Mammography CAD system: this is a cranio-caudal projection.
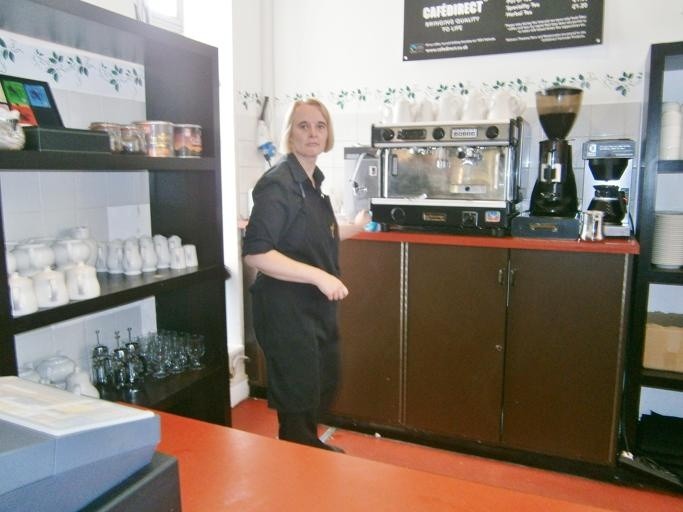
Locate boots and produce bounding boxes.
[276,409,346,455]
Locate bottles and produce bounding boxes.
[174,123,202,159]
[92,345,112,386]
[65,366,100,399]
[71,227,99,267]
[125,341,149,384]
[121,124,147,153]
[138,122,175,157]
[18,361,41,383]
[112,348,133,389]
[91,122,122,152]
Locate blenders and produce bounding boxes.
[530,87,583,217]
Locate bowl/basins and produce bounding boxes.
[660,101,683,161]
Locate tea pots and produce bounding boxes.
[168,235,186,268]
[106,239,122,274]
[122,239,142,277]
[52,236,89,267]
[8,272,38,317]
[96,241,107,273]
[33,265,69,308]
[184,243,199,266]
[12,240,52,272]
[153,234,169,269]
[139,234,157,273]
[66,259,101,300]
[6,249,16,274]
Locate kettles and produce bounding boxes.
[36,351,76,384]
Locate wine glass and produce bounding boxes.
[139,331,206,380]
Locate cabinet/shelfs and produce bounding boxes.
[619,39,683,489]
[239,227,401,440]
[401,244,633,481]
[0,0,232,430]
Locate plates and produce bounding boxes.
[652,210,683,270]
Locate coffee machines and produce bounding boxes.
[581,139,633,237]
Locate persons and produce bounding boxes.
[242,97,372,454]
